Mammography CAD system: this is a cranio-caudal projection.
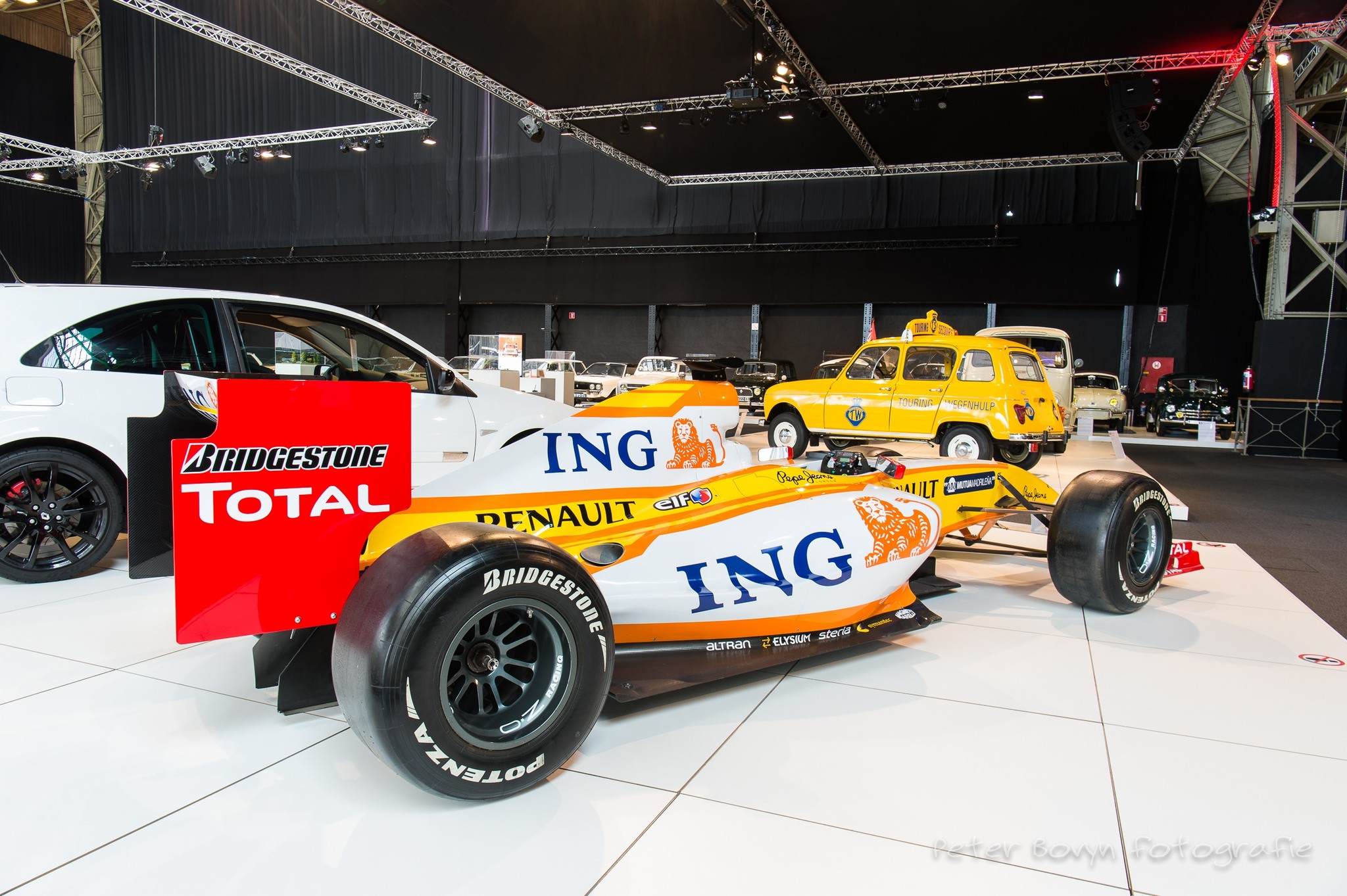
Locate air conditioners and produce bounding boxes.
[907,91,924,111]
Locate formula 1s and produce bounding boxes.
[124,379,1206,802]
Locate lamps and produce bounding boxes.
[863,94,888,115]
[1244,41,1267,77]
[238,148,249,163]
[226,150,237,164]
[778,102,794,119]
[339,134,384,153]
[754,32,809,95]
[805,99,825,120]
[642,115,657,130]
[1251,206,1278,221]
[517,114,545,143]
[700,109,714,128]
[193,151,217,179]
[937,89,949,109]
[1275,42,1293,67]
[254,144,291,158]
[727,107,751,127]
[1028,81,1044,99]
[619,115,630,134]
[679,109,694,126]
[561,120,575,136]
[0,125,175,192]
[418,129,437,145]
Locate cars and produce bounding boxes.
[1146,372,1236,440]
[762,309,1127,472]
[0,279,595,588]
[357,347,798,411]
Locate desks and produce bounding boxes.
[1232,397,1342,460]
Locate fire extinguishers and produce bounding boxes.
[1139,401,1146,417]
[1243,366,1254,390]
